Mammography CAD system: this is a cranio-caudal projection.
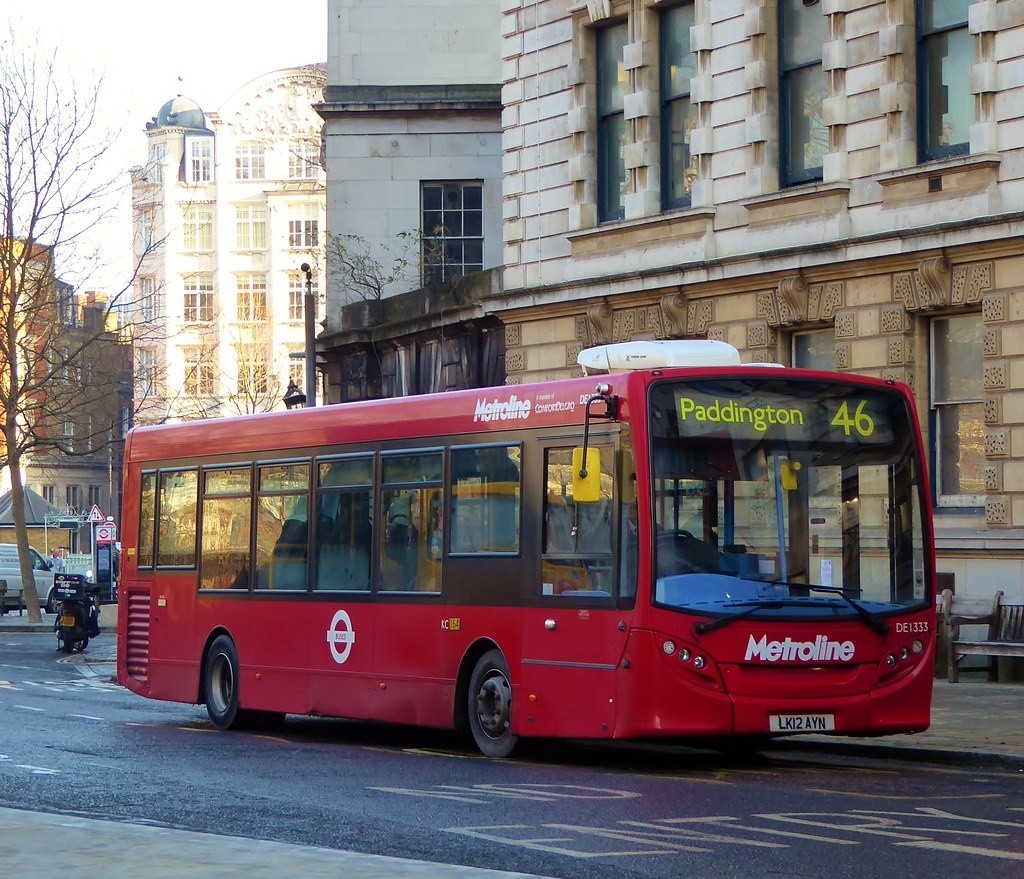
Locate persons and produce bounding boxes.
[44,547,63,574]
[609,498,664,550]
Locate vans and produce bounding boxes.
[0,542,65,614]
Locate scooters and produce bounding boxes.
[53,579,100,652]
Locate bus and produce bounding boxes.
[114,339,939,764]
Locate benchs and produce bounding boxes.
[945,590,1024,684]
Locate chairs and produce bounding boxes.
[248,511,423,592]
